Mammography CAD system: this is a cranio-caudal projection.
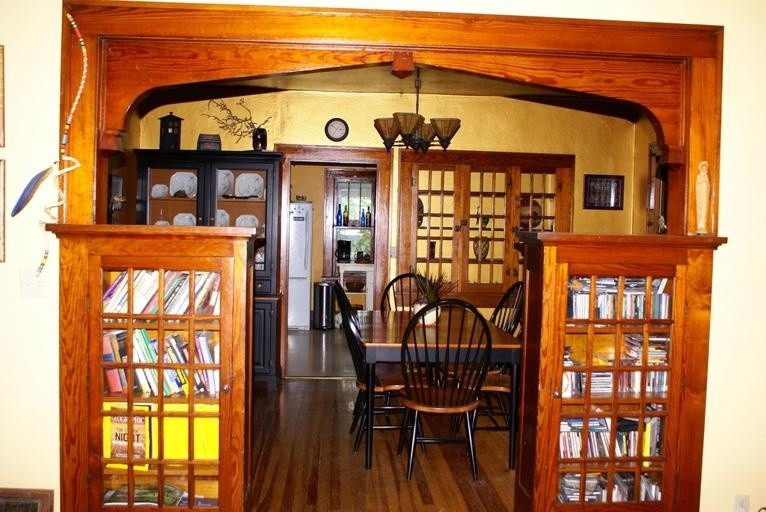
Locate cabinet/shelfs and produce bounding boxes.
[514,229,728,511]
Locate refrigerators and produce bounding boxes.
[288,201,313,331]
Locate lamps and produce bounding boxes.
[373,68,461,153]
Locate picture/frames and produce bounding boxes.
[583,174,624,211]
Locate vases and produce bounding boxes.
[252,128,267,150]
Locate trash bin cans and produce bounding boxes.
[313,282,336,331]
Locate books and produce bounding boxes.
[95,262,219,507]
[556,270,668,504]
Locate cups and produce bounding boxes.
[354,251,363,261]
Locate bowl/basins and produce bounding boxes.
[296,195,307,201]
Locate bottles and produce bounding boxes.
[366,206,371,227]
[343,205,349,226]
[364,255,370,261]
[337,204,342,225]
[361,208,365,227]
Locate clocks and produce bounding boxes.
[324,118,349,142]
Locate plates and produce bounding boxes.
[150,183,168,199]
[169,172,197,198]
[235,214,259,227]
[235,173,264,200]
[216,169,234,197]
[216,209,229,227]
[155,220,170,225]
[173,213,196,226]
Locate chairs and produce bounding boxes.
[332,273,524,482]
